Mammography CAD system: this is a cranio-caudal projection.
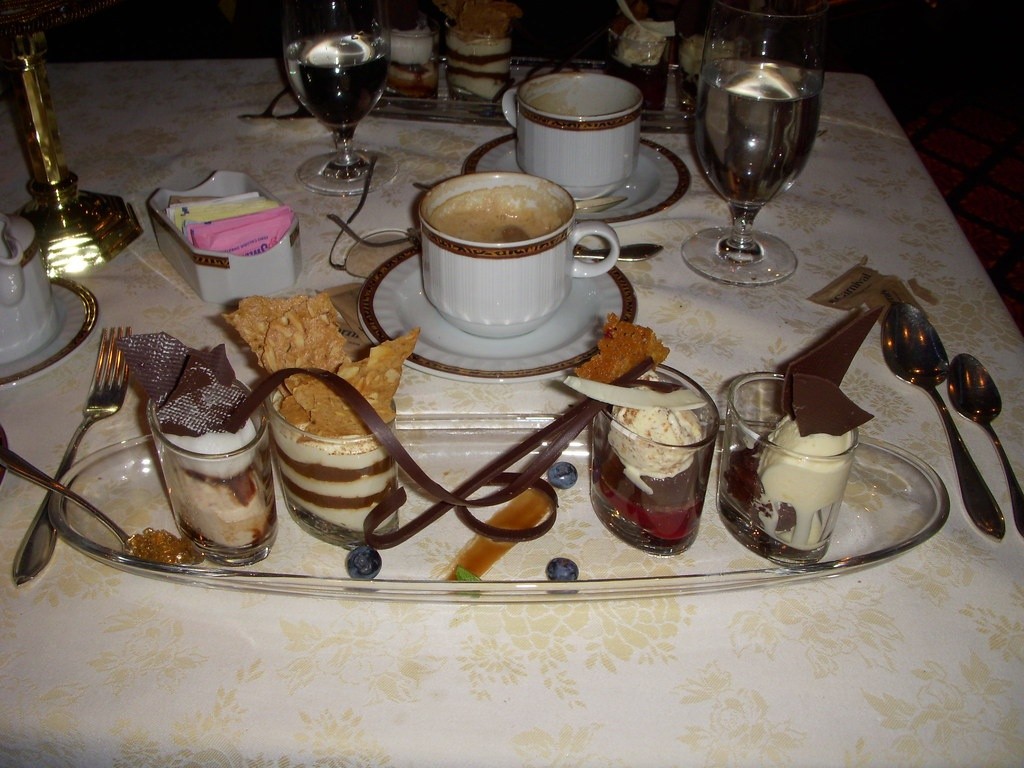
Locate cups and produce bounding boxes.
[714,372,859,567]
[606,26,673,110]
[265,393,399,548]
[589,362,719,556]
[385,21,440,97]
[0,210,62,366]
[501,70,641,202]
[417,174,622,338]
[444,16,512,103]
[146,378,277,558]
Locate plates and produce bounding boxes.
[358,246,638,384]
[0,278,99,392]
[461,132,691,225]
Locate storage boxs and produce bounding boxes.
[147,170,300,304]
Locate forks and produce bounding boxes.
[12,326,134,587]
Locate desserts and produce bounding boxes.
[376,0,743,113]
[116,300,887,567]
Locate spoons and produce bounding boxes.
[0,446,204,565]
[880,301,1004,541]
[238,86,316,122]
[947,353,1024,538]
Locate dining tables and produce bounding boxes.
[0,59,1024,768]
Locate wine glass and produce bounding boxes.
[682,0,834,287]
[281,0,400,196]
